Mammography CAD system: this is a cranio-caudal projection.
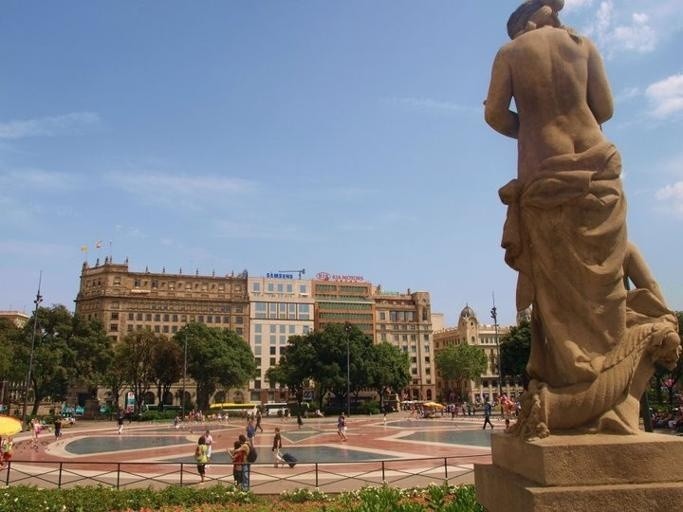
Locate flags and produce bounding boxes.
[80,245,87,253]
[95,241,101,248]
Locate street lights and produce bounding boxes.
[182,333,194,418]
[21,270,45,434]
[489,288,504,417]
[344,322,352,418]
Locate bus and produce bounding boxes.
[264,402,290,417]
[144,404,183,417]
[209,403,257,416]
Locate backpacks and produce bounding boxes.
[242,443,257,462]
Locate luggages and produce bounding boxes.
[279,451,297,468]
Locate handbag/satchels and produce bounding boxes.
[195,454,203,461]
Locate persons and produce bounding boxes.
[69,412,75,425]
[483,399,494,429]
[648,404,683,432]
[483,1,664,383]
[135,400,149,412]
[338,411,348,441]
[227,434,251,487]
[31,418,62,440]
[196,431,212,482]
[495,394,516,427]
[383,404,477,418]
[272,427,286,467]
[1,435,12,469]
[118,408,132,433]
[239,409,264,444]
[281,408,321,427]
[175,408,229,424]
